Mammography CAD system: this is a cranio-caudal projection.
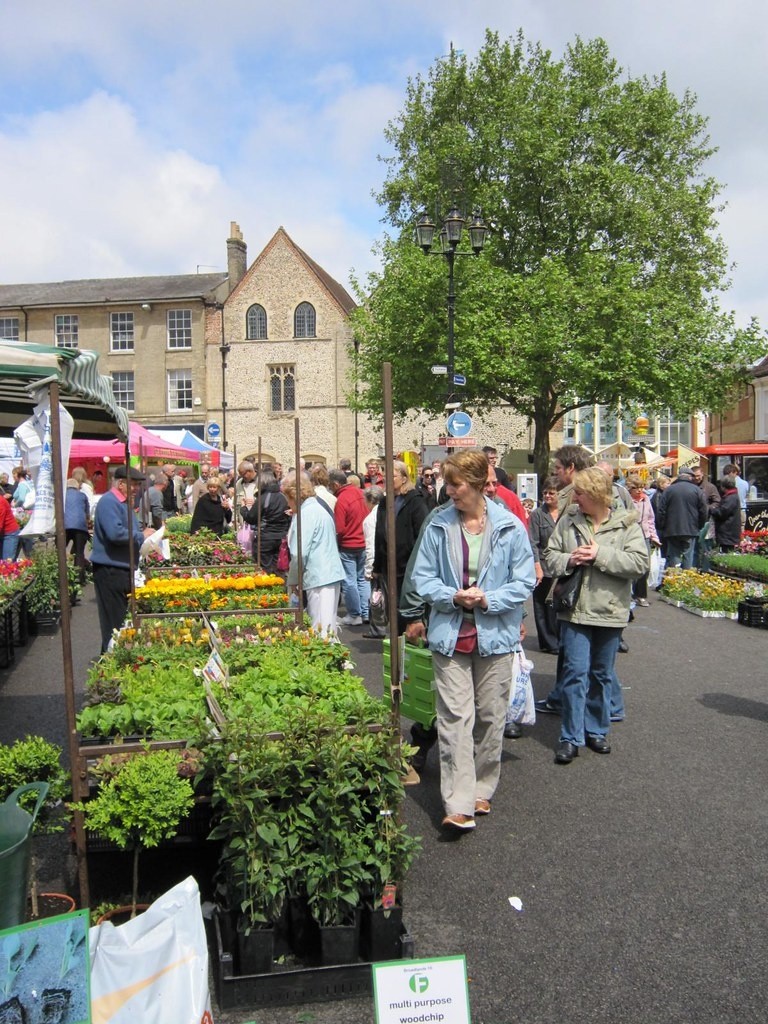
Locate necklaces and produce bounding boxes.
[461,499,486,535]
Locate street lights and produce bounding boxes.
[414,187,490,451]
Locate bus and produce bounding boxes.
[668,444,768,536]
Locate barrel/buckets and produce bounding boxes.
[0,781,50,930]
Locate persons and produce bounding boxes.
[0,445,750,777]
[410,449,537,827]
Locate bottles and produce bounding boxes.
[750,485,757,499]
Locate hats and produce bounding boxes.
[679,467,695,476]
[114,466,147,481]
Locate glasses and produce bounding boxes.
[542,491,557,495]
[633,486,644,490]
[423,474,432,478]
[488,456,498,459]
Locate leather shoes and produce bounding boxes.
[584,735,611,753]
[555,742,578,762]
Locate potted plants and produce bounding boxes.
[31,546,75,634]
[0,737,193,928]
[220,723,409,975]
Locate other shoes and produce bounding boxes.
[504,722,522,738]
[547,648,559,654]
[635,598,649,606]
[618,641,629,652]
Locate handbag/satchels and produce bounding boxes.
[646,545,666,588]
[505,642,536,726]
[705,515,716,539]
[367,575,389,637]
[552,526,584,611]
[277,538,291,571]
[237,520,254,555]
[22,482,35,508]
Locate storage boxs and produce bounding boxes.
[738,601,764,626]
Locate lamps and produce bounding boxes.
[142,303,151,313]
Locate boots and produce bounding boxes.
[407,722,438,769]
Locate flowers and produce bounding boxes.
[660,566,767,619]
[734,530,768,552]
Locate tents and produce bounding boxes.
[663,444,768,482]
[0,338,236,911]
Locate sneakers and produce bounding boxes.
[341,614,363,625]
[610,705,624,721]
[534,700,562,714]
[441,812,476,828]
[474,798,490,813]
[363,617,369,623]
